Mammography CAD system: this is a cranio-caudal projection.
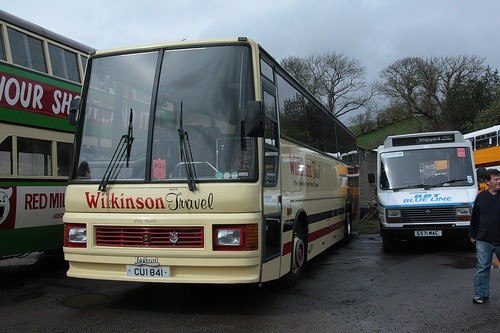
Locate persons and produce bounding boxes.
[468,169,500,303]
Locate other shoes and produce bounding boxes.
[492,259,499,268]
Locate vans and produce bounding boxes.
[368,131,478,249]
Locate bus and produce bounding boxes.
[63,36,358,290]
[0,12,95,260]
[462,124,500,194]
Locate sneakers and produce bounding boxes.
[472,295,489,304]
